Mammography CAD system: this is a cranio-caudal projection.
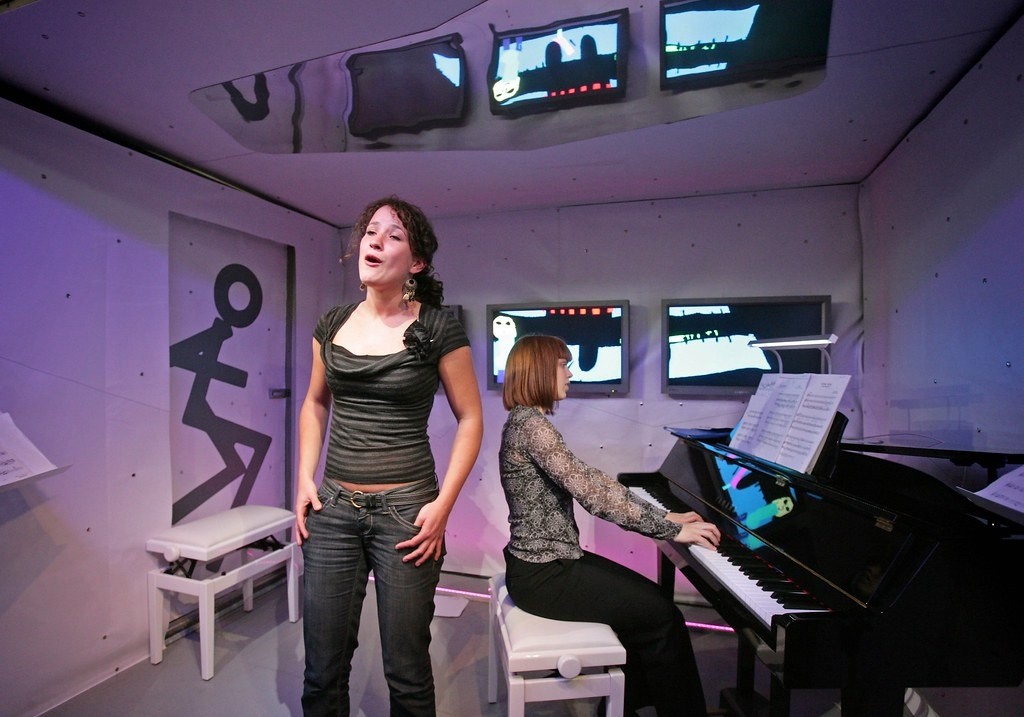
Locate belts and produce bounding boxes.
[322,478,440,508]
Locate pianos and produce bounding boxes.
[618,413,1024,717]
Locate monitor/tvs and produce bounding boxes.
[659,0,834,91]
[488,8,629,117]
[662,295,831,396]
[487,299,630,393]
[346,32,467,135]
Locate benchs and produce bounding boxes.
[145,505,300,680]
[487,571,627,717]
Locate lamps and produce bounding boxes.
[748,334,838,374]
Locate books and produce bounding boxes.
[728,373,853,474]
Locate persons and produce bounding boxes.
[291,198,485,717]
[498,335,722,717]
